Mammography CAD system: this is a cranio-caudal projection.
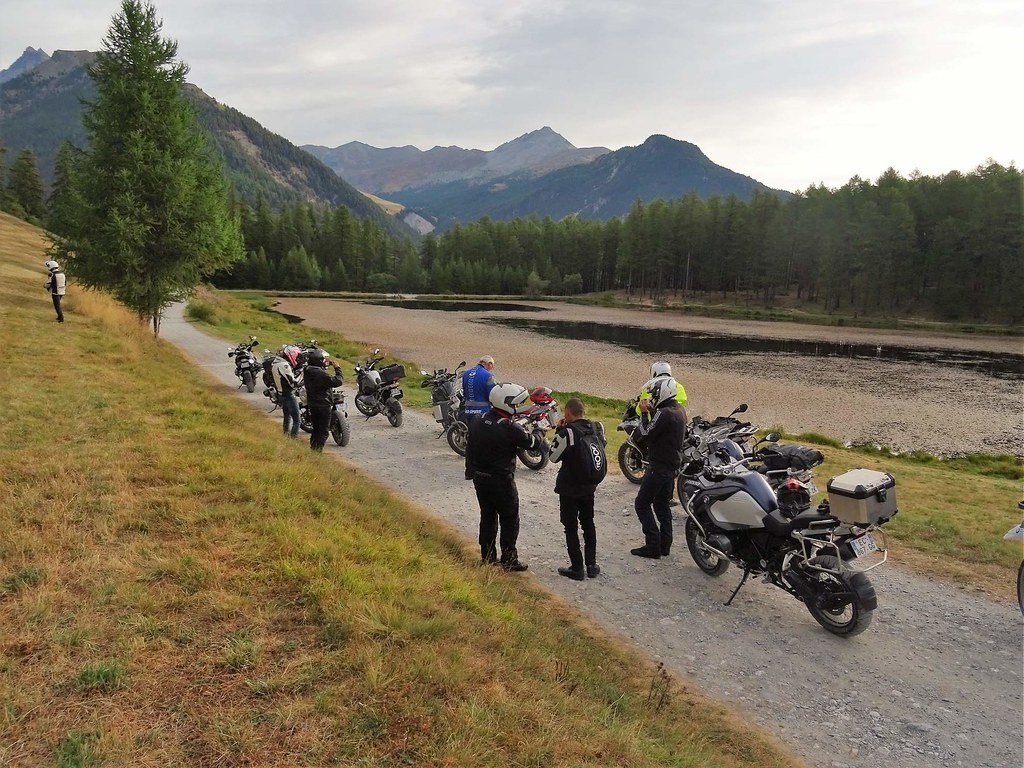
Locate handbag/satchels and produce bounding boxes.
[756,443,824,471]
[431,381,453,401]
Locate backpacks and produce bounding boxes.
[569,421,607,486]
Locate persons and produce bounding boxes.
[304,349,342,451]
[42,262,67,323]
[461,355,495,479]
[465,382,552,571]
[549,398,607,581]
[635,361,687,507]
[271,345,302,439]
[631,378,688,558]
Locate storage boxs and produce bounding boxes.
[434,401,449,423]
[827,468,898,528]
[379,364,405,381]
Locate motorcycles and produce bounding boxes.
[616,393,762,485]
[352,348,406,429]
[420,361,561,472]
[673,430,900,638]
[259,339,351,447]
[226,335,261,394]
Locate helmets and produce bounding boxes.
[647,377,677,409]
[46,261,59,271]
[530,386,555,405]
[489,382,529,414]
[307,349,329,367]
[777,479,811,517]
[650,361,672,378]
[285,346,302,367]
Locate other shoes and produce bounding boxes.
[661,544,670,555]
[558,566,585,580]
[587,564,600,577]
[668,499,678,506]
[482,559,501,564]
[503,561,528,571]
[631,545,661,558]
